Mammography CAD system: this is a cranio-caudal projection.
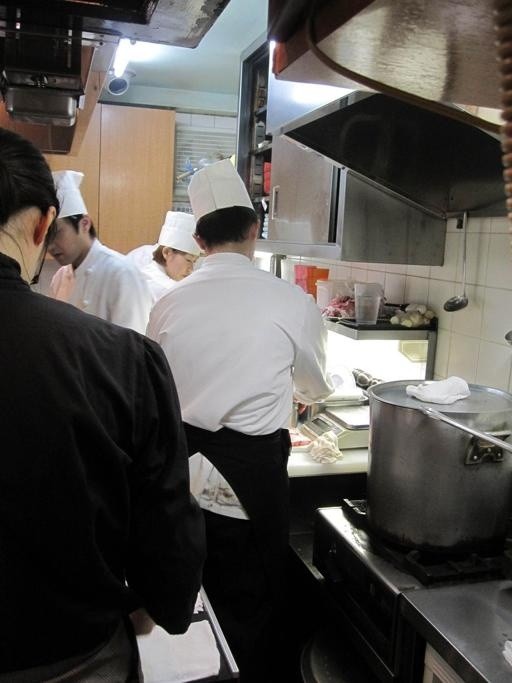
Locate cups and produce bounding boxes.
[353,282,381,326]
[305,266,330,301]
[293,264,317,293]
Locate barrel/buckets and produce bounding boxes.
[317,277,353,313]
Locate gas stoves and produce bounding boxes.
[342,498,512,589]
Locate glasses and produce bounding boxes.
[28,226,50,284]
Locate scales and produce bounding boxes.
[300,404,369,448]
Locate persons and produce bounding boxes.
[145,240,200,322]
[148,206,335,682]
[0,123,208,682]
[44,209,147,342]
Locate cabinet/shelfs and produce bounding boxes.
[44,101,177,260]
[233,29,447,266]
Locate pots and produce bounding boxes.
[362,378,511,557]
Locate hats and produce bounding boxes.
[49,167,89,218]
[187,156,256,222]
[159,208,204,259]
